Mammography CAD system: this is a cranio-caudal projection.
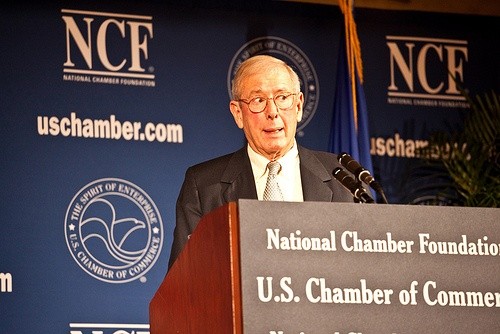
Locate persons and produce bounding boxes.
[167,56,373,272]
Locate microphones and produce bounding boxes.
[337,152,389,204]
[332,167,376,204]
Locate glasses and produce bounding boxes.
[234,93,299,114]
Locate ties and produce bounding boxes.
[263,162,284,201]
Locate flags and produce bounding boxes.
[325,0,376,200]
